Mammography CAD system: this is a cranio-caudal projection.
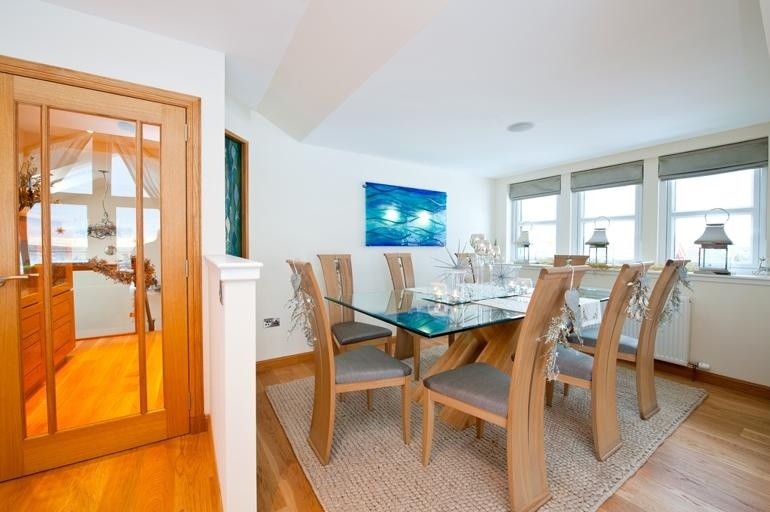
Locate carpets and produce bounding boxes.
[266,342,709,512]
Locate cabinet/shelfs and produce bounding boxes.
[21,264,76,402]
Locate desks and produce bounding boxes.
[321,277,614,442]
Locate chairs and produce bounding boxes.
[419,265,593,512]
[561,257,690,419]
[285,257,415,467]
[316,254,395,410]
[563,259,655,458]
[383,253,419,289]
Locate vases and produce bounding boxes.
[18,205,31,217]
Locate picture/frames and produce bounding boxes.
[225,130,249,260]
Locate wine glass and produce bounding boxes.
[469,233,500,266]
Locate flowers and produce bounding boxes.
[16,156,64,212]
[87,254,158,291]
